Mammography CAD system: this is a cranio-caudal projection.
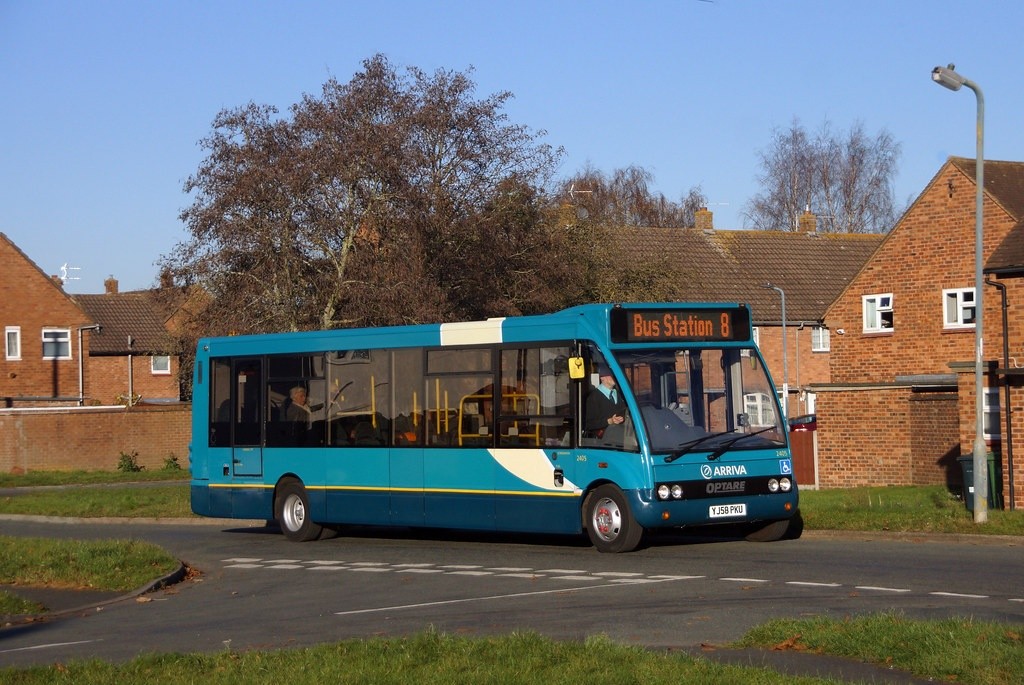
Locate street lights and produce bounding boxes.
[931,63,988,524]
[760,283,789,446]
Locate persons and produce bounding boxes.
[586,369,626,439]
[287,387,312,431]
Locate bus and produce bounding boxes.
[189,301,803,554]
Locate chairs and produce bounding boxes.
[217,398,479,448]
